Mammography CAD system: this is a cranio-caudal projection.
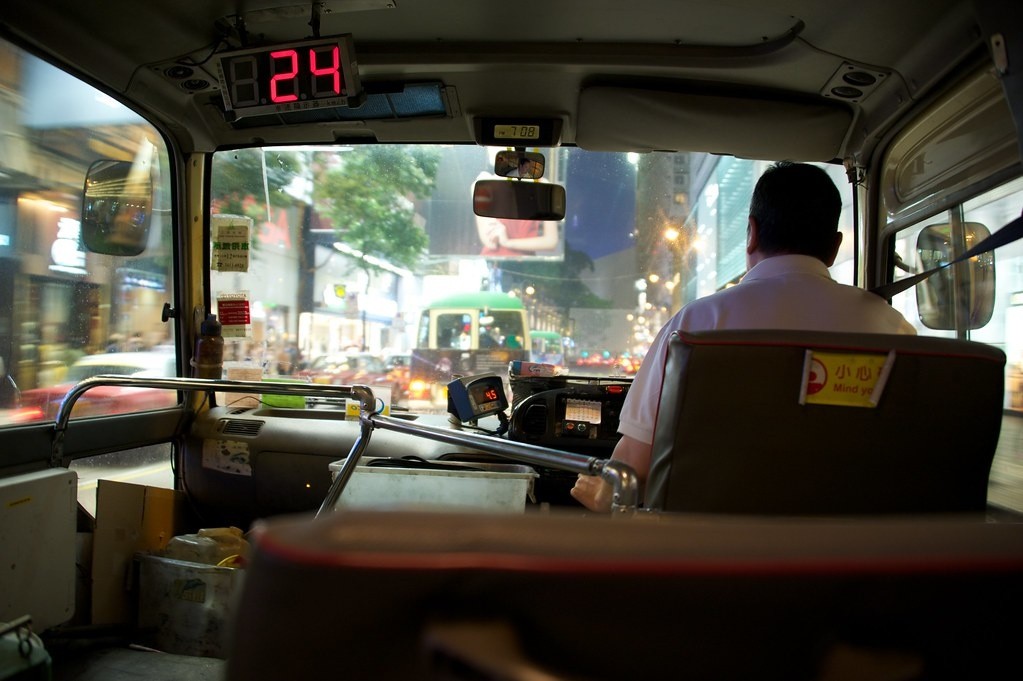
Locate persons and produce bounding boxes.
[472,147,559,256]
[568,161,916,514]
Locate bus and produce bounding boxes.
[415,297,530,384]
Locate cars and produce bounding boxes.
[385,354,437,400]
[296,352,399,405]
[18,352,179,423]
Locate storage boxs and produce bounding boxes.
[130,553,240,655]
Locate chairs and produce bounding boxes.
[219,508,1023,681]
[641,329,1007,519]
